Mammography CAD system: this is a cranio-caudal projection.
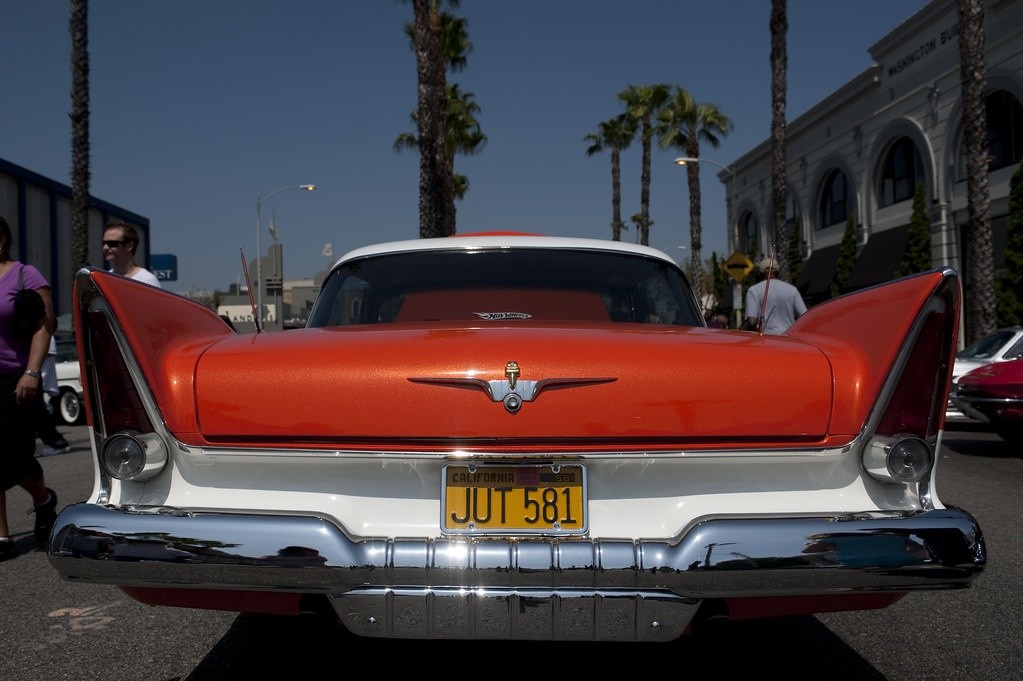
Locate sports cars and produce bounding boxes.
[48,233,988,643]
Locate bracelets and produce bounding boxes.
[25,370,40,378]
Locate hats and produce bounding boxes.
[758,258,780,275]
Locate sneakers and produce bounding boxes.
[0,536,18,562]
[26,487,58,542]
[41,438,71,456]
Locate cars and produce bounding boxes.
[952,325,1023,446]
[42,338,88,425]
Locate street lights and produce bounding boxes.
[256,184,319,333]
[673,156,742,332]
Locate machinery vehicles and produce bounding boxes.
[239,246,260,333]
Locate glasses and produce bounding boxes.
[102,240,128,248]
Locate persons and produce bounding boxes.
[0,216,71,561]
[705,309,729,329]
[102,222,161,288]
[743,258,807,336]
